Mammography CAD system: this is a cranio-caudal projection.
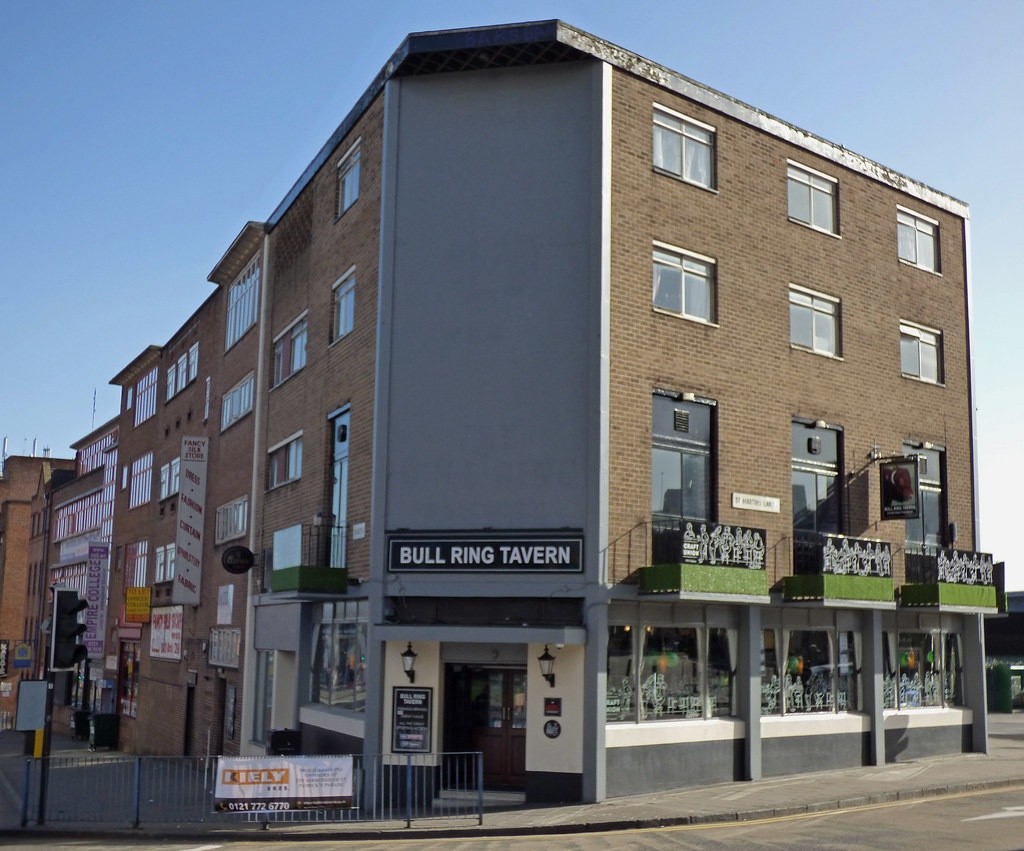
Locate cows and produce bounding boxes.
[882,465,913,506]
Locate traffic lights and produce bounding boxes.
[49,585,89,672]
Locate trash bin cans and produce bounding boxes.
[87,713,120,752]
[264,728,301,755]
[70,710,91,743]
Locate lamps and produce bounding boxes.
[538,644,555,687]
[401,641,417,683]
